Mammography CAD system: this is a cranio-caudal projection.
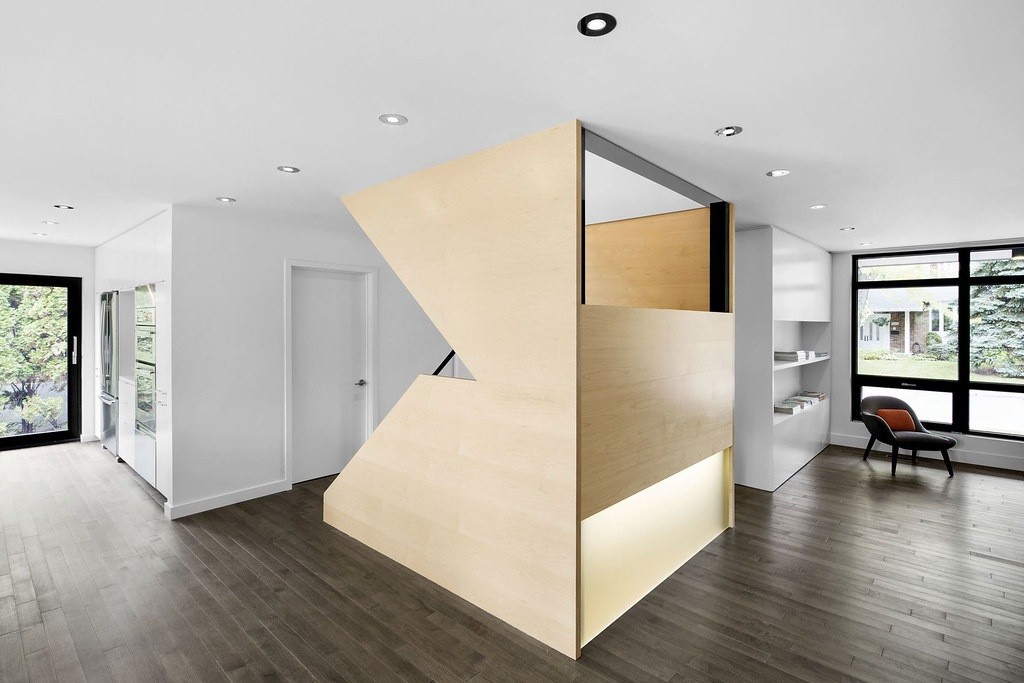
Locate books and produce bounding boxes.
[774,349,828,362]
[774,390,826,415]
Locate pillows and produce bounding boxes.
[878,409,916,431]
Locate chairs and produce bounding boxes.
[859,395,958,477]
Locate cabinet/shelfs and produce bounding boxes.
[734,226,833,493]
